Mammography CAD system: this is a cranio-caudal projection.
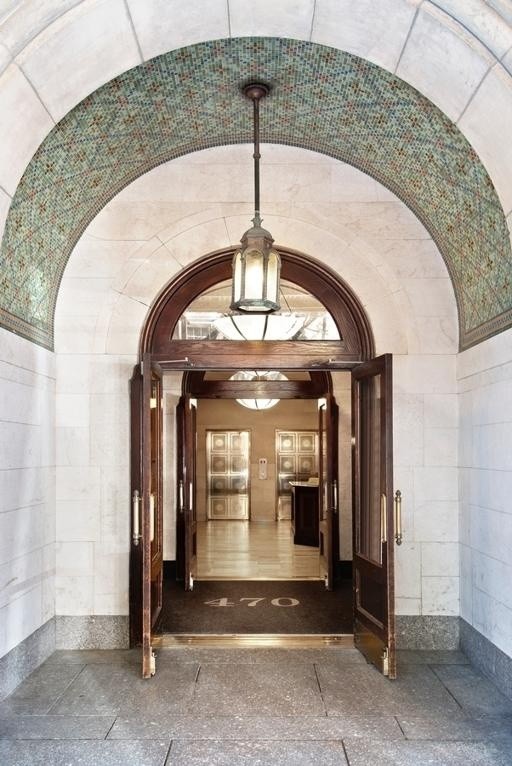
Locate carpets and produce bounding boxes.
[162,580,354,634]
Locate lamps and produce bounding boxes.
[230,82,282,315]
[211,280,307,412]
